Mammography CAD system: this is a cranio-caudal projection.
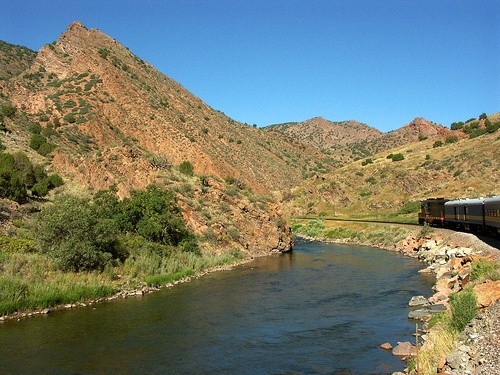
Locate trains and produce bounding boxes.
[416,196,500,239]
